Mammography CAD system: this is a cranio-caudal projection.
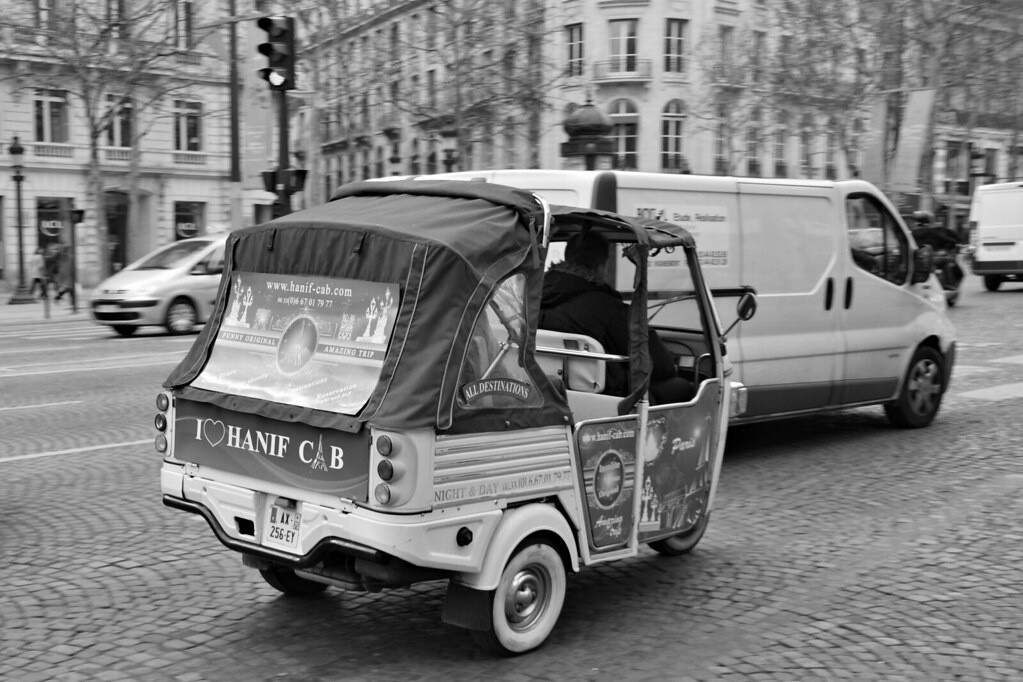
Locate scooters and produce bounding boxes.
[895,224,963,307]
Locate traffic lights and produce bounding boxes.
[257,18,295,89]
[283,169,303,188]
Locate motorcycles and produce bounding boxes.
[155,178,759,657]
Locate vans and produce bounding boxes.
[367,171,964,434]
[963,182,1023,290]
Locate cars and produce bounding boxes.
[847,227,907,273]
[92,234,228,336]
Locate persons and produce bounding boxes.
[910,210,954,290]
[28,248,50,299]
[526,227,685,413]
[932,213,966,288]
[48,246,78,311]
